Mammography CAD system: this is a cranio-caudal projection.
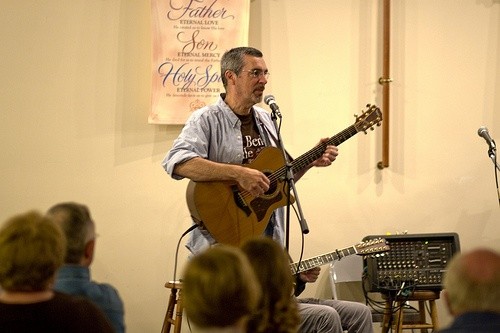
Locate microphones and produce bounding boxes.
[477,127,496,150]
[264,95,282,119]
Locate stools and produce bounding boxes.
[160,281,187,333]
[383,292,440,333]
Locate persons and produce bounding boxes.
[0,209,117,333]
[181,243,262,333]
[429,248,500,332]
[162,47,340,255]
[294,265,374,333]
[236,235,304,332]
[44,202,125,333]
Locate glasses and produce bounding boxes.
[233,69,269,77]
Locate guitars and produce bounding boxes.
[289,239,391,277]
[187,103,382,248]
